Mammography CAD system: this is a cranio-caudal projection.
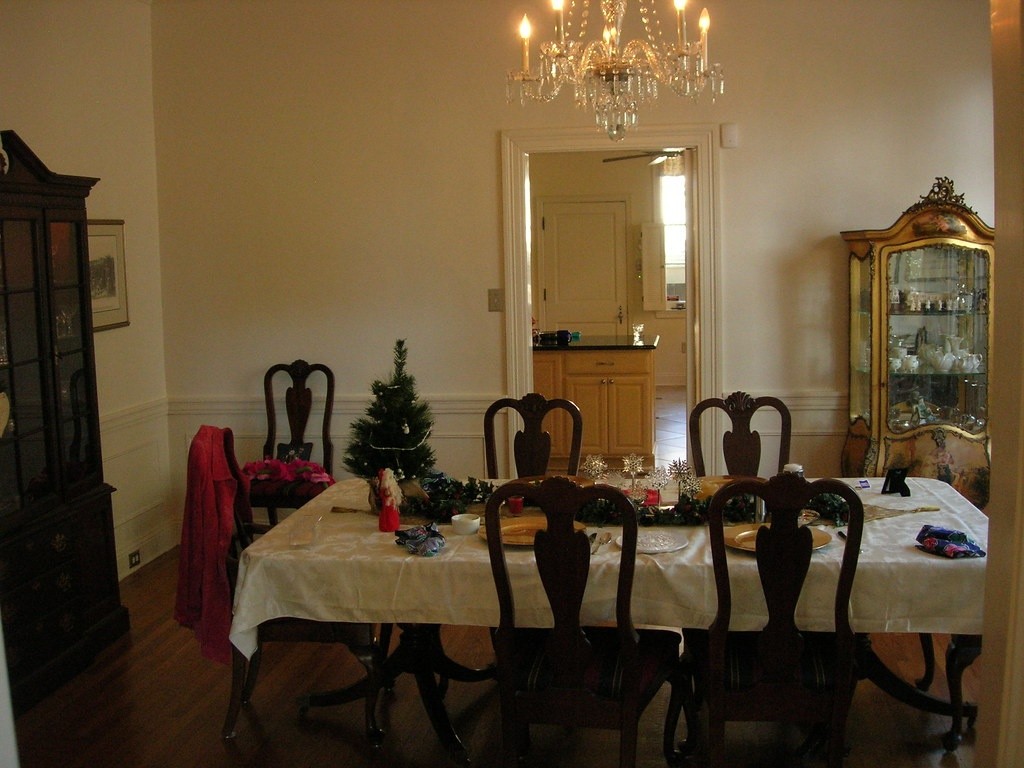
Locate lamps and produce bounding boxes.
[505,0,724,142]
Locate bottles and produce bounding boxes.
[783,463,804,479]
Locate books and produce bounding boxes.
[667,295,685,309]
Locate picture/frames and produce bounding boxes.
[87,219,130,332]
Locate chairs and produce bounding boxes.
[482,388,868,768]
[246,360,334,536]
[172,424,387,751]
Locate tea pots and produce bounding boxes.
[862,334,983,373]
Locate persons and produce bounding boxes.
[379,468,402,532]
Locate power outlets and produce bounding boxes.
[128,549,141,568]
[487,288,506,311]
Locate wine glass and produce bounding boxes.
[50,299,82,336]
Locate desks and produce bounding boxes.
[224,476,995,768]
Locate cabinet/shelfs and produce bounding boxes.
[838,174,996,511]
[0,128,134,723]
[533,349,656,483]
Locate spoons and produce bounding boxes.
[592,532,612,555]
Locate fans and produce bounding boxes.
[602,147,685,166]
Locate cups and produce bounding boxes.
[451,513,480,535]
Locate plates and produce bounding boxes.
[479,516,586,546]
[720,522,832,554]
[616,529,689,553]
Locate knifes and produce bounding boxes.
[586,533,597,546]
[838,529,848,541]
